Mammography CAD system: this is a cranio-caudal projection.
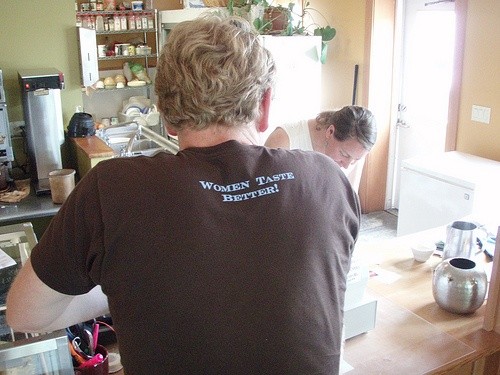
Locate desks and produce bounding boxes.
[0,179,63,223]
[344,219,500,375]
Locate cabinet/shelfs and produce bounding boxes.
[395,150,500,237]
[75,9,163,93]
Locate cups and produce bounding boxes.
[48,169,78,205]
[74,345,109,375]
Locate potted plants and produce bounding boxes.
[225,0,337,65]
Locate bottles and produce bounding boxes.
[82,12,154,31]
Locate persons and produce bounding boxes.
[263,106,378,194]
[6,10,360,375]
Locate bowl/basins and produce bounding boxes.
[412,244,434,264]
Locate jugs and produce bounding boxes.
[442,221,486,261]
